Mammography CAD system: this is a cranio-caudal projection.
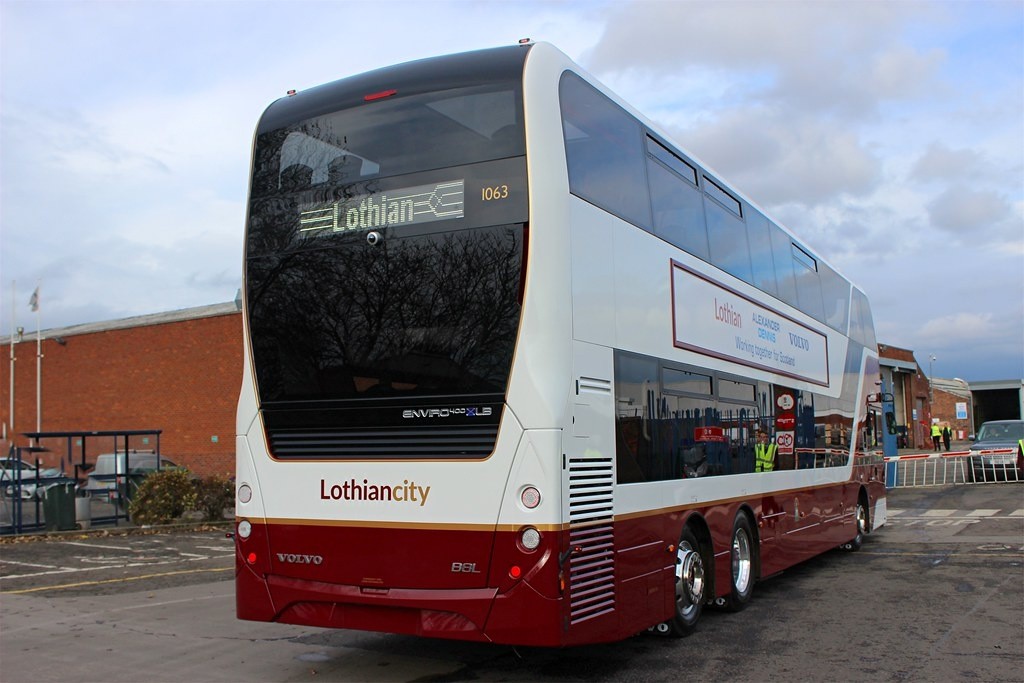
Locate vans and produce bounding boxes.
[94,448,179,502]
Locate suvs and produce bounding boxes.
[966,420,1024,482]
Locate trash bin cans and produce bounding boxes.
[40,477,82,534]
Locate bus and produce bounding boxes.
[235,39,897,648]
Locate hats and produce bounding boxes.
[944,422,947,424]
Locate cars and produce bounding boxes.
[0,457,43,490]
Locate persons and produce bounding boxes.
[985,427,1002,438]
[928,422,942,452]
[940,422,953,451]
[751,431,780,525]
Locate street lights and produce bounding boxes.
[929,354,936,421]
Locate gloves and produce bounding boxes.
[931,436,932,439]
